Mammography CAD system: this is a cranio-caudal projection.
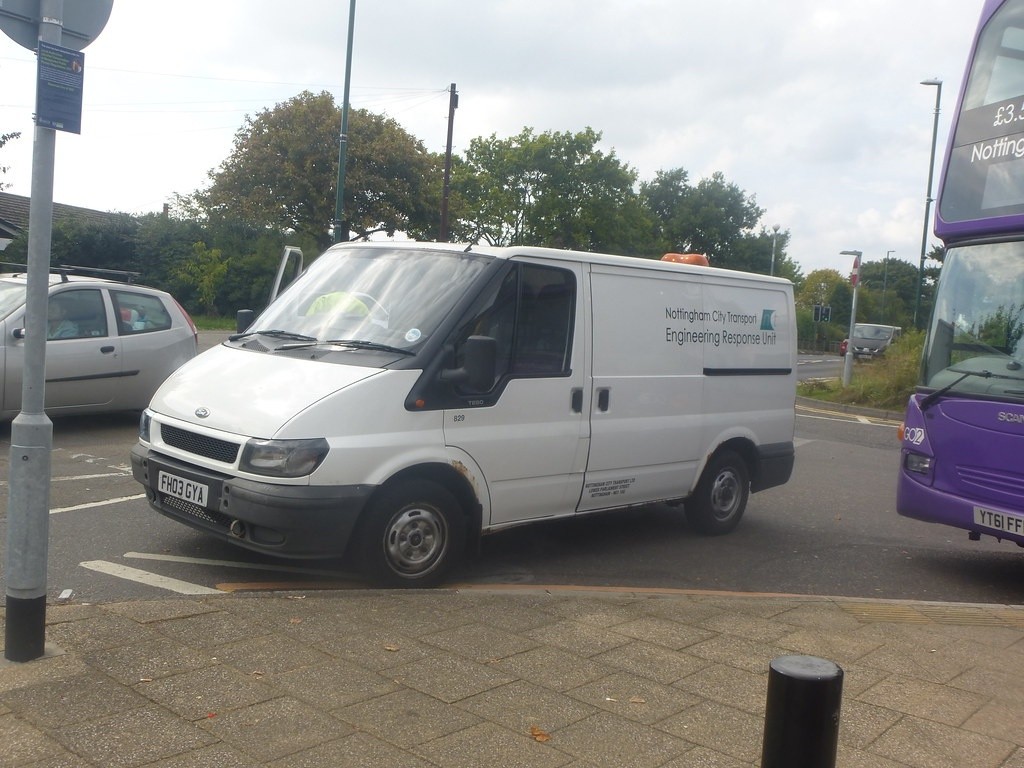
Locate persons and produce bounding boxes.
[46,297,81,339]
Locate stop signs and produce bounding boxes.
[849,257,860,287]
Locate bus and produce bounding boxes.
[897,0,1024,546]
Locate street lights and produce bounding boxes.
[839,249,863,391]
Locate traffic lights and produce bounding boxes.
[813,305,820,322]
[823,307,831,323]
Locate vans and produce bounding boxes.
[131,239,797,583]
[0,265,200,425]
[848,324,902,366]
